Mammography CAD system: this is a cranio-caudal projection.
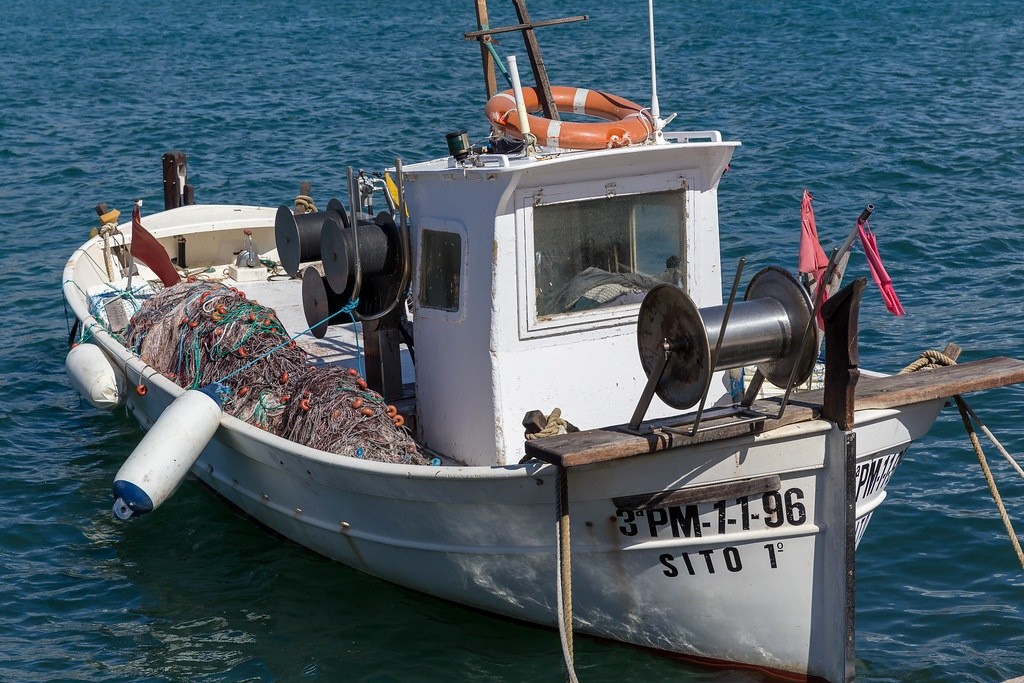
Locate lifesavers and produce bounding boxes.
[484,85,654,148]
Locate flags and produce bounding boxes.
[128,200,182,288]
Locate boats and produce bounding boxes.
[61,0,957,683]
